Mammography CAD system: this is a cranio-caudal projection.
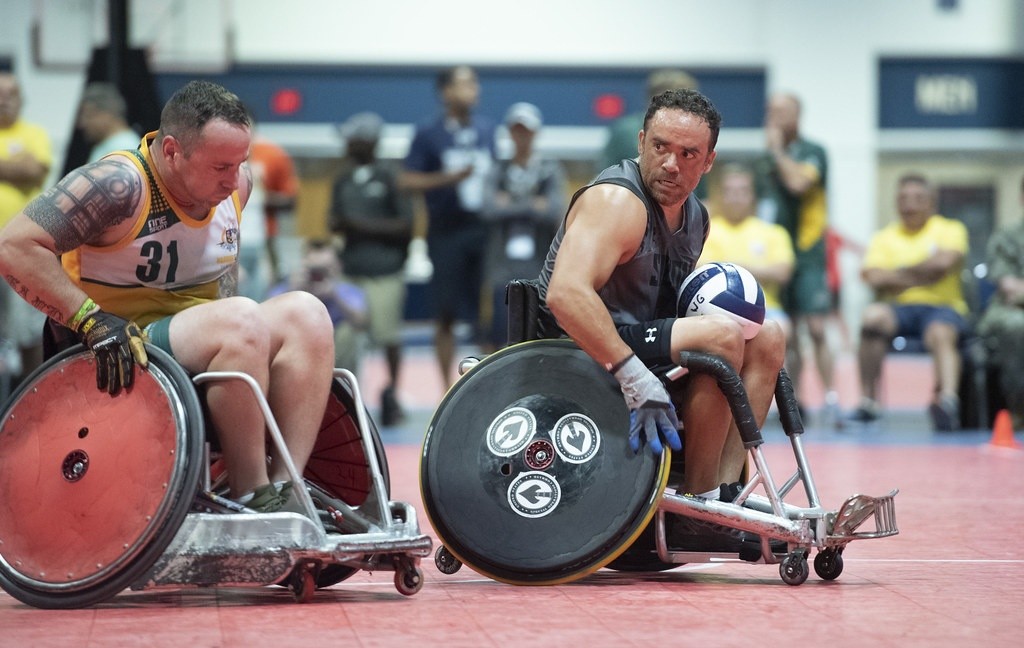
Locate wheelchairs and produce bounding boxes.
[0,333,433,610]
[418,279,900,586]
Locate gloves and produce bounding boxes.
[608,355,682,456]
[68,300,152,394]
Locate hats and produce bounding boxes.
[504,101,543,136]
[339,114,384,144]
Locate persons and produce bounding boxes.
[755,94,831,425]
[696,163,795,344]
[326,110,414,426]
[842,176,969,431]
[591,69,700,173]
[827,228,858,336]
[974,176,1024,432]
[537,89,788,553]
[0,55,51,377]
[248,115,298,283]
[481,101,564,352]
[392,64,498,392]
[1,80,334,513]
[76,83,140,165]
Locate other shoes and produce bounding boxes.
[846,396,883,426]
[379,403,402,428]
[931,393,961,431]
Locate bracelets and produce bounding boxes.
[70,298,96,331]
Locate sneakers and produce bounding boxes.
[230,478,341,528]
[674,484,790,551]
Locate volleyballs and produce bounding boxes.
[676,259,768,345]
